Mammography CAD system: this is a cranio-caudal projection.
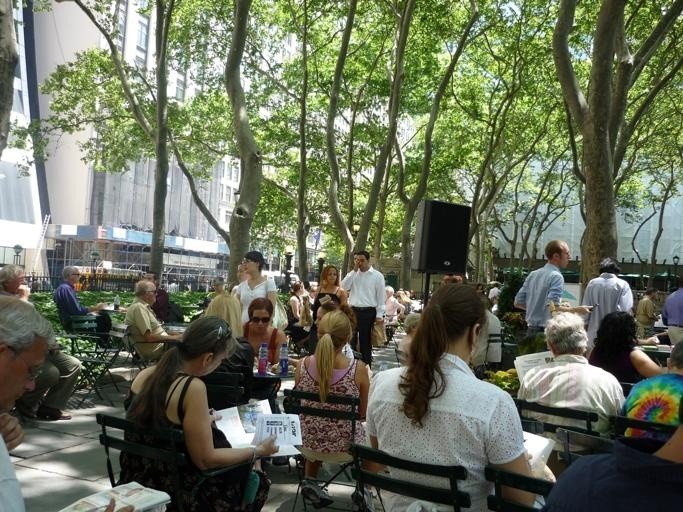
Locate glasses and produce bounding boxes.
[147,290,157,294]
[9,346,43,380]
[250,316,271,323]
[242,259,257,265]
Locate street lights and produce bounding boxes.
[90,251,99,272]
[283,246,292,292]
[13,245,22,266]
[317,252,325,285]
[672,254,678,291]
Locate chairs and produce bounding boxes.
[62,308,681,512]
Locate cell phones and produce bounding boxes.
[589,304,599,311]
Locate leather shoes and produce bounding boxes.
[14,399,37,419]
[36,404,72,420]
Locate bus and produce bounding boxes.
[619,272,681,306]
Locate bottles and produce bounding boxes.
[277,343,288,377]
[114,294,119,310]
[257,342,268,376]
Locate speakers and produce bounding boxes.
[411,199,472,274]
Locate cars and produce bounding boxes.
[199,281,213,292]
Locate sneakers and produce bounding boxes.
[301,479,334,510]
[350,486,376,512]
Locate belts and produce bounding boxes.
[667,324,683,328]
[528,326,545,332]
[351,306,377,311]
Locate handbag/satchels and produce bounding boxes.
[264,275,289,332]
